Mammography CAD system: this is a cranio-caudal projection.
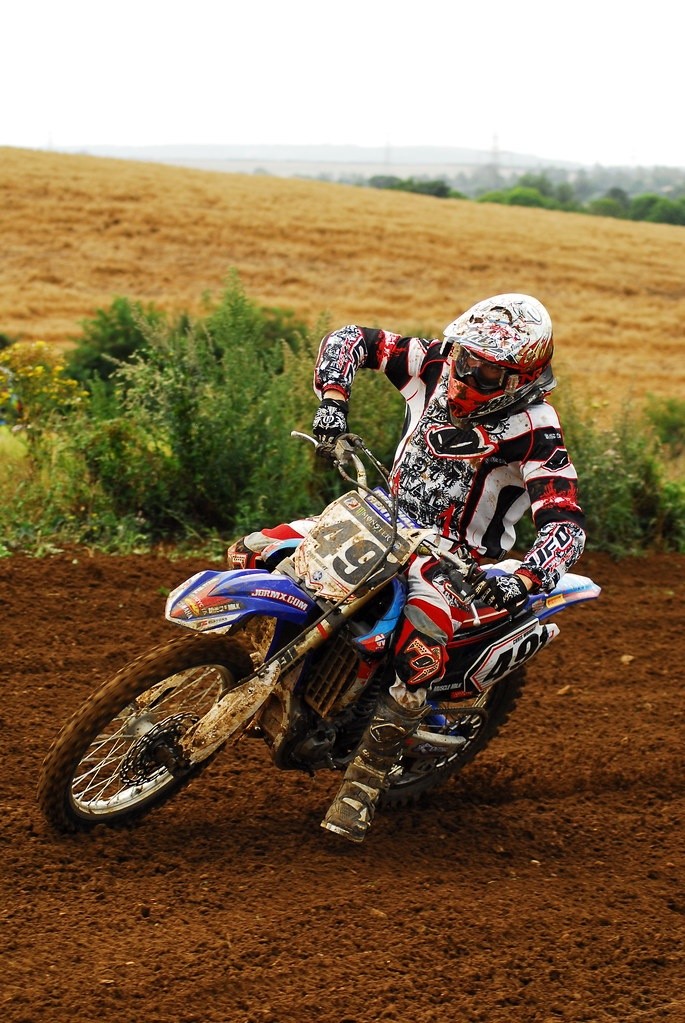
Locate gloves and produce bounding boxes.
[312,398,357,448]
[473,574,528,612]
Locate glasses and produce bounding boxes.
[454,344,509,392]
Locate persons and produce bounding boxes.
[227,293,587,843]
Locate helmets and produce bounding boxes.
[443,294,558,431]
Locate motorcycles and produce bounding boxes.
[35,432,603,837]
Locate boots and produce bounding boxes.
[320,688,433,843]
